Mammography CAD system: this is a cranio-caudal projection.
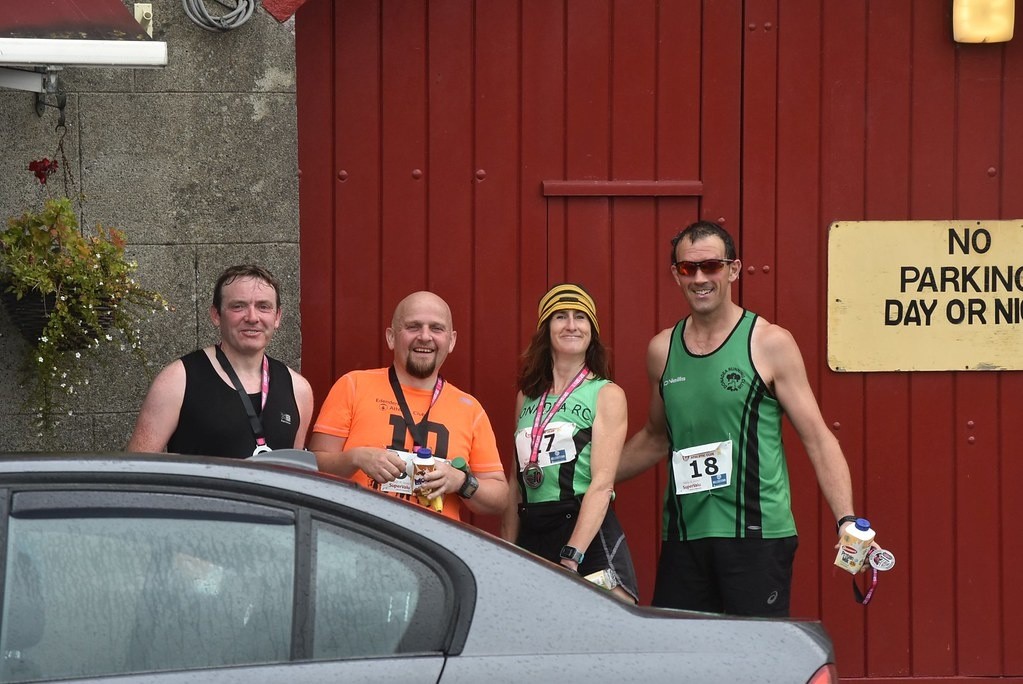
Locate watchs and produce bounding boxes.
[560,545,584,564]
[457,471,479,500]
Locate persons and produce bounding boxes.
[500,284,639,605]
[308,291,510,522]
[614,220,882,617]
[128,265,313,453]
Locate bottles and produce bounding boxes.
[582,568,617,592]
[411,448,435,497]
[833,517,876,576]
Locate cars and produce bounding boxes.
[0,447,841,684]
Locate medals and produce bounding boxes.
[253,446,272,456]
[523,464,544,489]
[869,549,895,571]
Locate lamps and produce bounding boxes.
[948,0,1017,47]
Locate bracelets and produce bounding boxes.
[836,515,859,534]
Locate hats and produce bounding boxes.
[536,284,600,338]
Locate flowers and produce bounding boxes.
[0,157,178,445]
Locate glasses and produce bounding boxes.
[674,258,734,277]
